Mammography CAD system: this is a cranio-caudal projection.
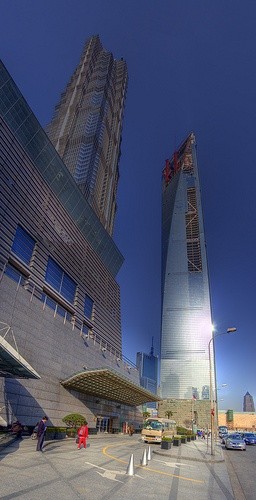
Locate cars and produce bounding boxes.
[218,425,256,451]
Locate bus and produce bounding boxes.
[139,417,177,444]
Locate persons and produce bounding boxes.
[201,431,206,439]
[77,421,89,450]
[10,420,24,440]
[36,415,48,451]
[131,423,134,430]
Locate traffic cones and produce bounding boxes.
[139,448,148,467]
[147,445,152,461]
[125,454,135,476]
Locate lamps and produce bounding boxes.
[102,349,106,351]
[128,367,131,369]
[83,335,88,339]
[117,358,120,361]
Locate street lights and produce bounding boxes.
[192,397,203,434]
[207,328,238,456]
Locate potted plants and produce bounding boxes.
[162,438,172,449]
[46,414,86,440]
[173,435,196,446]
